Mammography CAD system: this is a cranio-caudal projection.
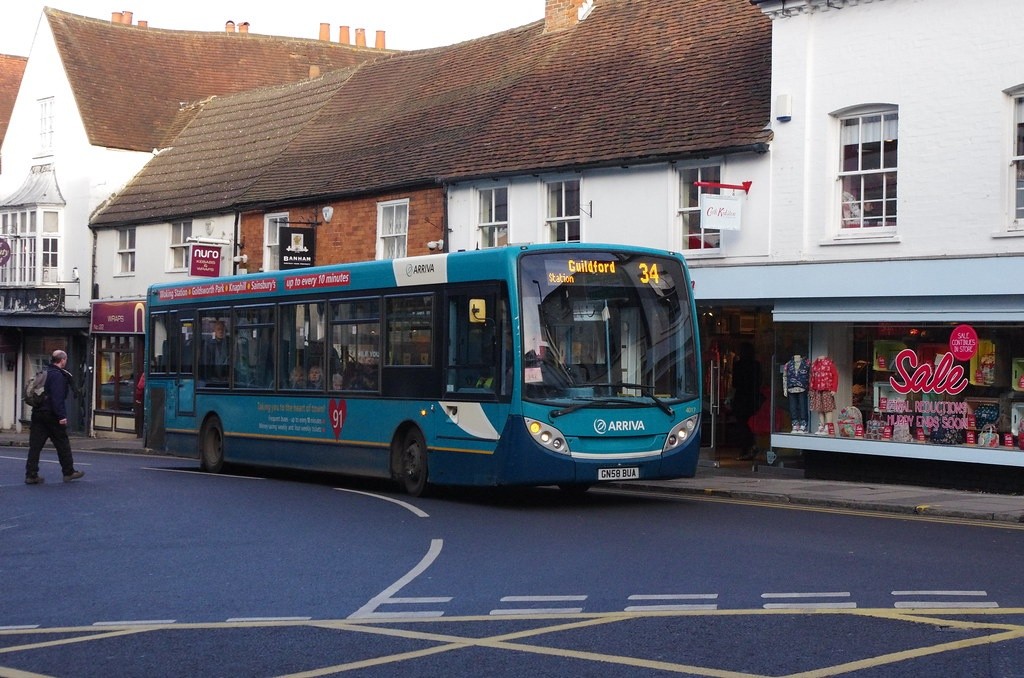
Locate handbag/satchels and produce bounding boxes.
[866,409,889,439]
[978,424,1000,448]
[894,417,912,442]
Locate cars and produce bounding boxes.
[98,378,135,412]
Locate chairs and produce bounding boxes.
[162,334,357,389]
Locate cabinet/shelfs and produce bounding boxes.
[873,338,1024,436]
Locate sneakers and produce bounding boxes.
[791,426,799,434]
[63,470,84,482]
[798,425,809,433]
[25,477,45,484]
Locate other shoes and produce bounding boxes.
[815,427,823,434]
[822,428,829,435]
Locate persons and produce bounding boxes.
[332,368,346,390]
[344,365,364,390]
[731,345,764,459]
[306,365,323,390]
[318,338,339,376]
[468,324,514,391]
[288,365,308,389]
[859,196,886,221]
[25,349,86,484]
[809,356,838,436]
[206,320,230,379]
[783,354,812,433]
[136,359,155,452]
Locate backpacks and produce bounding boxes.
[24,369,59,407]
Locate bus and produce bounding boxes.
[143,241,704,500]
